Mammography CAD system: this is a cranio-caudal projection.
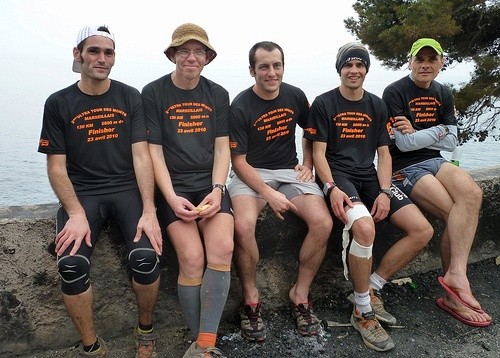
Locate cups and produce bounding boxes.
[450,159,459,167]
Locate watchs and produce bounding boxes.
[378,189,393,200]
[322,182,337,198]
[212,184,226,196]
[440,124,449,135]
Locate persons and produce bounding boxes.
[37,24,164,358]
[140,22,236,358]
[227,42,334,341]
[304,43,434,351]
[383,38,493,326]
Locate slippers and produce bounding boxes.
[438,276,483,314]
[437,297,491,327]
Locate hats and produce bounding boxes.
[73,24,115,73]
[164,22,217,67]
[336,42,370,77]
[409,38,444,57]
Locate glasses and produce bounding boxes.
[175,49,206,57]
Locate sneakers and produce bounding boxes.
[292,302,321,335]
[132,320,159,358]
[61,337,110,358]
[239,299,267,340]
[182,341,224,358]
[348,286,397,327]
[350,306,395,351]
